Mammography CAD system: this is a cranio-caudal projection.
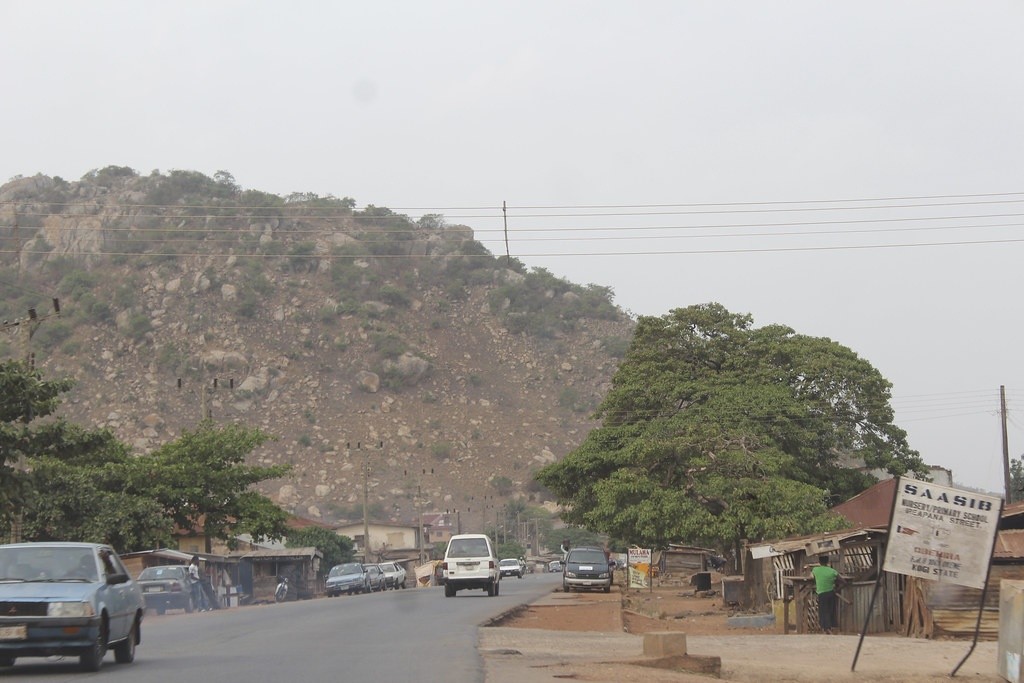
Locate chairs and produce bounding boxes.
[8,564,30,580]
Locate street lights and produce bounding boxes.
[404,468,435,566]
[346,441,384,562]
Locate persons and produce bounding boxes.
[519,557,525,575]
[188,556,213,612]
[811,556,839,634]
[63,555,99,581]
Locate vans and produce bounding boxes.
[442,533,503,597]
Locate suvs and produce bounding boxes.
[559,545,616,593]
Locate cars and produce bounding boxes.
[135,564,198,615]
[498,558,523,579]
[549,561,562,573]
[0,542,148,673]
[324,562,371,597]
[362,562,387,593]
[380,561,408,590]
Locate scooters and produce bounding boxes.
[519,563,527,576]
[273,573,292,604]
